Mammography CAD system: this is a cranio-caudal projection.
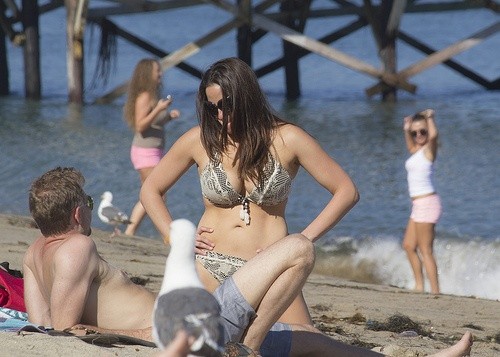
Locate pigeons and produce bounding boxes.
[96,191,132,239]
[152,218,230,357]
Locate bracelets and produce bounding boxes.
[426,116,433,119]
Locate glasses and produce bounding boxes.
[411,129,426,136]
[79,195,93,210]
[206,95,232,114]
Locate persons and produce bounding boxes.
[139,58,360,327]
[24,167,474,357]
[403,109,443,297]
[154,330,196,357]
[123,58,180,246]
